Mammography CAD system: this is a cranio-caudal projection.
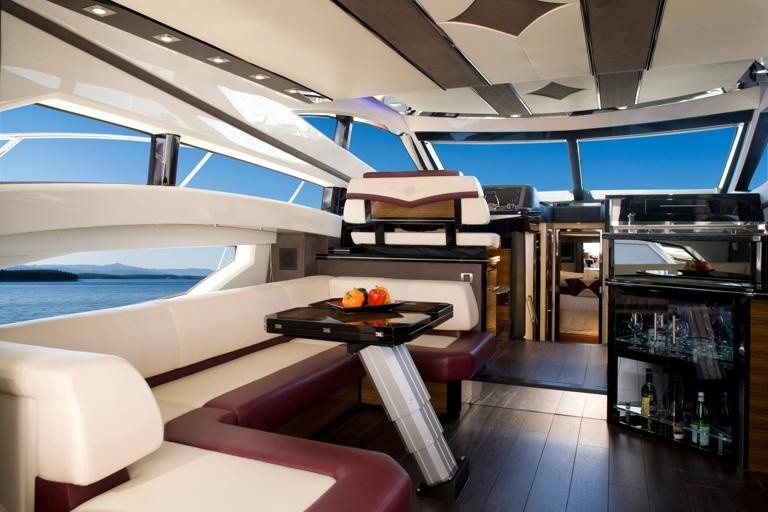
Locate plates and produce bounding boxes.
[325,299,405,312]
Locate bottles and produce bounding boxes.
[638,368,734,450]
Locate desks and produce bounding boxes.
[264,297,470,506]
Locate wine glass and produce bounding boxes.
[628,310,729,353]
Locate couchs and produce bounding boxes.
[0,274,497,511]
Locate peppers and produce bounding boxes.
[342,285,390,308]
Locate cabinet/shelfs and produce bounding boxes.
[601,231,768,471]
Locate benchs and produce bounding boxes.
[344,169,503,248]
